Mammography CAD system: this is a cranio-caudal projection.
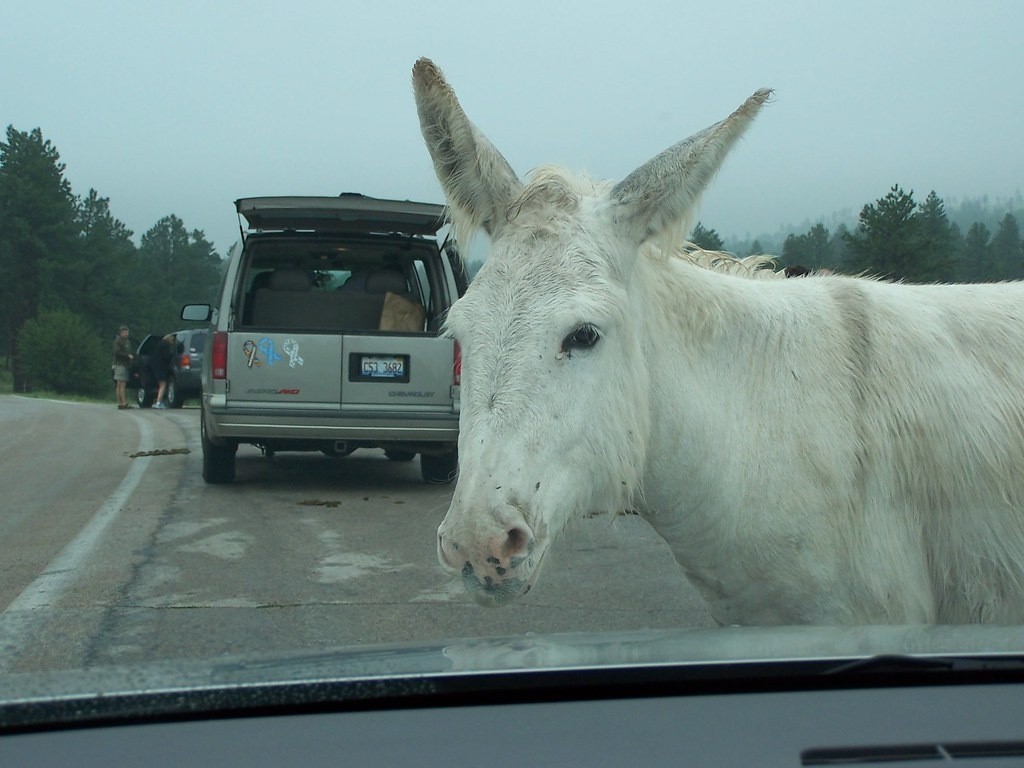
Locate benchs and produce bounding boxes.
[248,261,418,332]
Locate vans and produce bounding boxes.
[179,192,473,487]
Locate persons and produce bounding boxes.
[112,326,175,409]
[785,265,830,278]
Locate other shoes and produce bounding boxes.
[119,403,134,409]
[155,402,166,409]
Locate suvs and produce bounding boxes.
[111,326,210,409]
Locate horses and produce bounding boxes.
[411,57,1024,629]
